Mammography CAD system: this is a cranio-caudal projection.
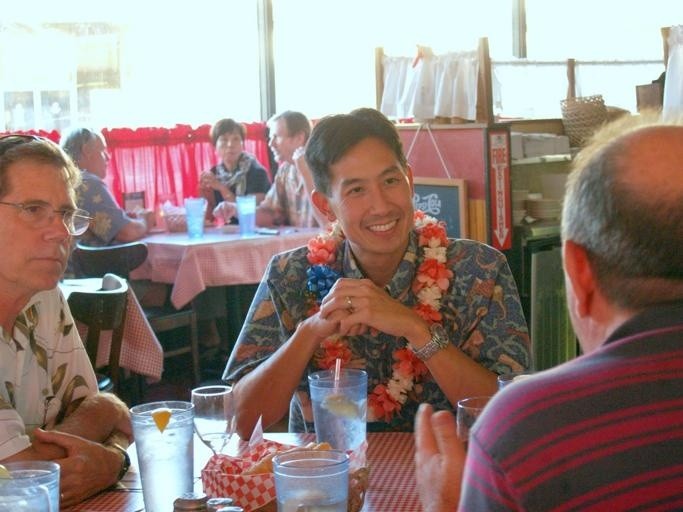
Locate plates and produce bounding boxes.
[510,186,563,228]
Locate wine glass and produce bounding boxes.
[189,383,238,457]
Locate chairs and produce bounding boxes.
[68,240,201,396]
[64,269,130,408]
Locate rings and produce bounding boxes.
[343,294,353,311]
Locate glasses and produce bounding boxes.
[1,200,91,236]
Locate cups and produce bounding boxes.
[0,459,60,511]
[495,371,535,388]
[130,400,198,512]
[510,131,570,162]
[456,395,493,453]
[270,447,351,512]
[185,196,205,238]
[307,367,367,478]
[235,194,256,238]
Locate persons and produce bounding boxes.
[222,108,530,443]
[0,134,132,512]
[411,123,681,512]
[211,111,340,234]
[196,114,271,236]
[54,124,158,279]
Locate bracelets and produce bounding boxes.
[103,438,131,491]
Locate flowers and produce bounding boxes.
[305,208,454,424]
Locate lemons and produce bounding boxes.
[321,394,359,417]
[151,408,170,432]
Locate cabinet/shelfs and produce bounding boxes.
[498,238,579,371]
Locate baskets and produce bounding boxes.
[165,215,187,232]
[561,95,609,147]
[252,460,369,512]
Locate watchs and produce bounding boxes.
[411,321,449,361]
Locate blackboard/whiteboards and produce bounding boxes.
[412,178,467,239]
